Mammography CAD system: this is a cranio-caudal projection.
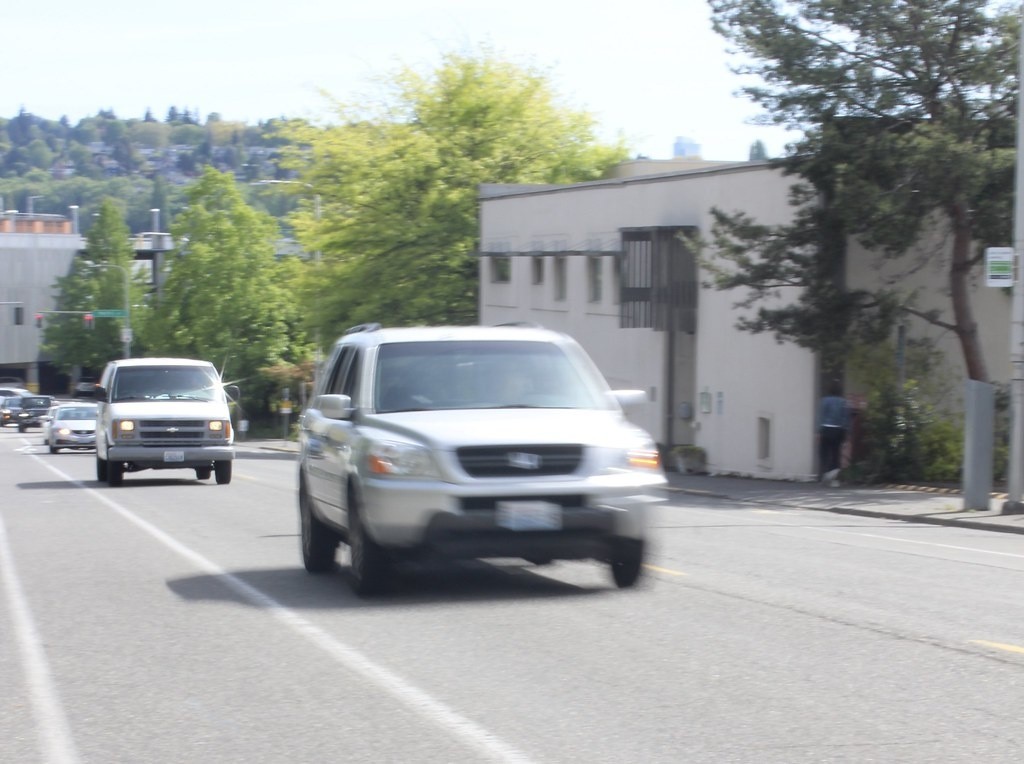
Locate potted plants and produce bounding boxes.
[671,446,704,474]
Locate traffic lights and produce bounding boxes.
[35,315,43,328]
[85,314,93,329]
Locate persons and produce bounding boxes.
[815,379,848,488]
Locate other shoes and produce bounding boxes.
[830,480,841,488]
[824,468,840,479]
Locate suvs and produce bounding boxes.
[299,322,667,596]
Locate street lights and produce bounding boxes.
[262,179,321,262]
[83,261,131,357]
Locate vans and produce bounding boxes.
[94,358,240,486]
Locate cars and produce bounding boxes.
[0,397,22,427]
[17,397,59,433]
[40,402,97,454]
[0,386,31,405]
